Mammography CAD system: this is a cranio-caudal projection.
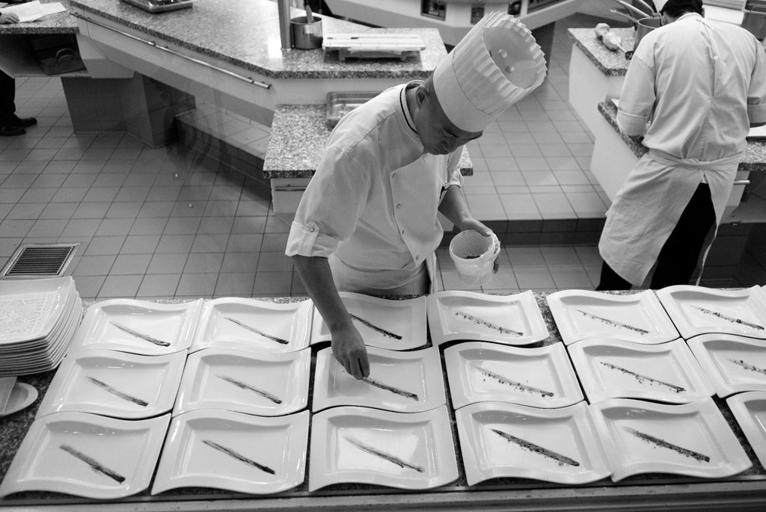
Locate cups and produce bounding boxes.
[449,227,499,285]
[289,14,324,50]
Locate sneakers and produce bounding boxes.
[0,114,38,136]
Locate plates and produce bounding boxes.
[0,274,84,380]
[444,342,588,413]
[307,405,463,493]
[654,284,764,343]
[1,410,172,501]
[33,348,190,420]
[1,375,40,419]
[171,348,313,416]
[422,289,551,347]
[685,332,765,399]
[725,389,765,471]
[65,296,204,358]
[452,400,614,489]
[567,340,710,405]
[312,293,429,352]
[189,295,316,355]
[585,400,755,483]
[545,288,683,345]
[150,407,310,500]
[314,345,446,416]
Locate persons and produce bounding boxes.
[595,0,766,291]
[285,73,501,381]
[0,8,37,138]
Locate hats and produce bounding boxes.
[432,9,549,133]
